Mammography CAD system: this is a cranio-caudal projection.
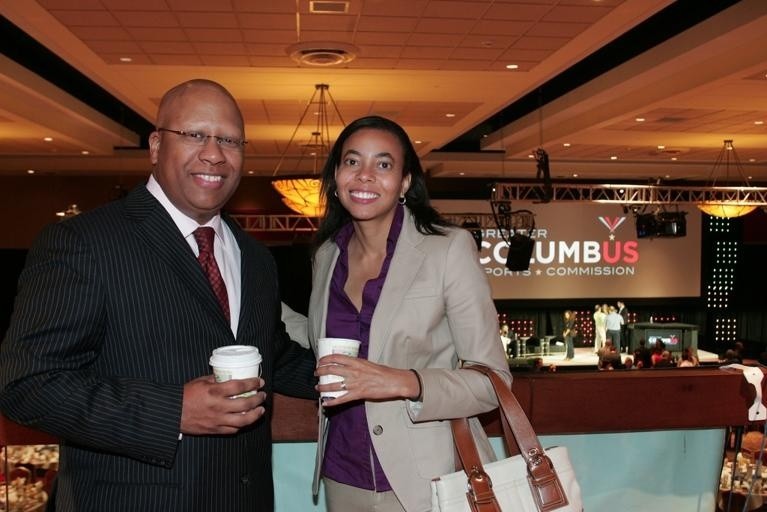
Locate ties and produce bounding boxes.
[192,226,230,322]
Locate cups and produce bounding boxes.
[209,344,263,401]
[315,338,363,399]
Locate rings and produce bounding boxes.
[340,381,347,390]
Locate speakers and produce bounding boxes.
[508,358,542,367]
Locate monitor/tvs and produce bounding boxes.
[645,329,683,352]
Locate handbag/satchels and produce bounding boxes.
[431,365,584,512]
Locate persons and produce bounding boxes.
[281,115,514,512]
[499,299,744,372]
[0,78,318,512]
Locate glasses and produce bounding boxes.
[157,126,248,150]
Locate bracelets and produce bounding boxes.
[410,369,422,402]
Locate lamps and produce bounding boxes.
[692,139,766,219]
[270,85,353,206]
[279,131,328,218]
